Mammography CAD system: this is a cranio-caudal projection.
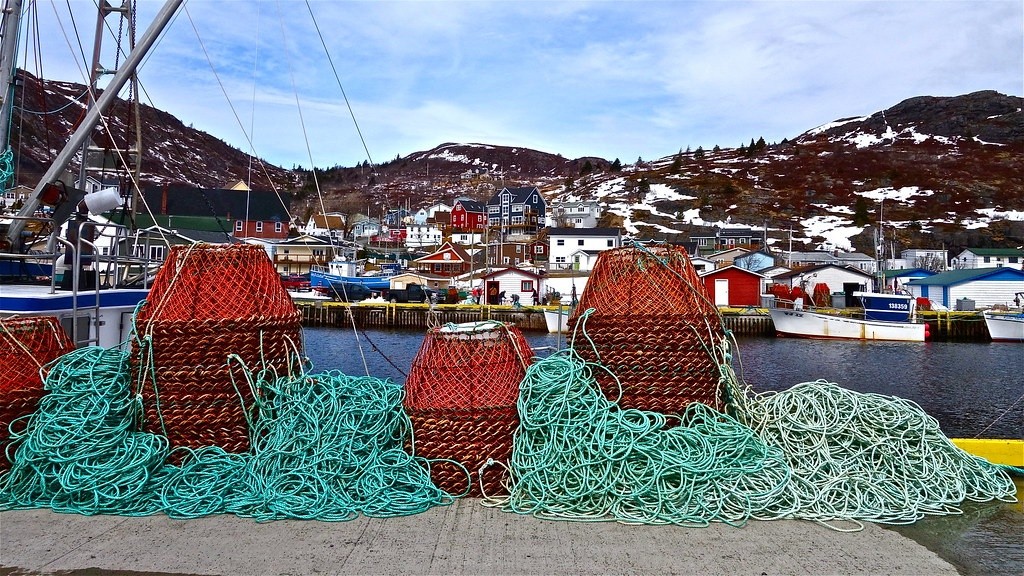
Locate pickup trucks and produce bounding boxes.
[382,283,446,303]
[278,272,312,292]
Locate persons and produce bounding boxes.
[498,290,506,305]
[489,286,497,304]
[510,294,519,305]
[476,289,481,305]
[530,288,540,305]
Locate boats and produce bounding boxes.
[542,307,570,334]
[982,309,1024,342]
[310,264,390,296]
[768,297,931,342]
